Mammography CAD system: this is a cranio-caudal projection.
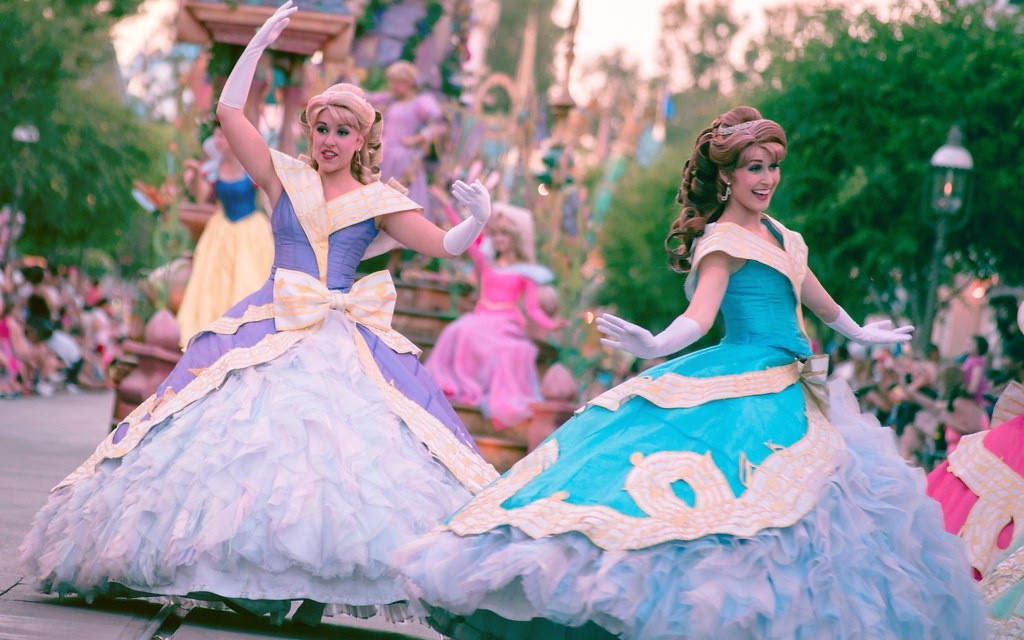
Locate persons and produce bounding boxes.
[20,0,505,627]
[139,252,194,315]
[0,255,133,400]
[390,105,995,639]
[978,534,1024,640]
[364,61,451,272]
[819,332,990,470]
[427,182,569,433]
[177,118,275,352]
[927,290,1024,582]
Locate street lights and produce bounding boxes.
[918,124,979,361]
